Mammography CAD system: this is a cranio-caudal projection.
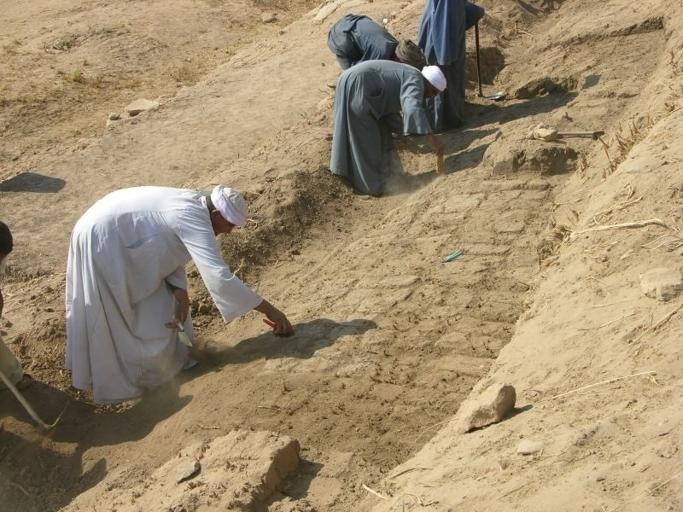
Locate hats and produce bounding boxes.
[421,64,446,93]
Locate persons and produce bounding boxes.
[326,13,426,74]
[0,221,35,394]
[418,1,485,133]
[64,187,293,402]
[328,58,448,196]
